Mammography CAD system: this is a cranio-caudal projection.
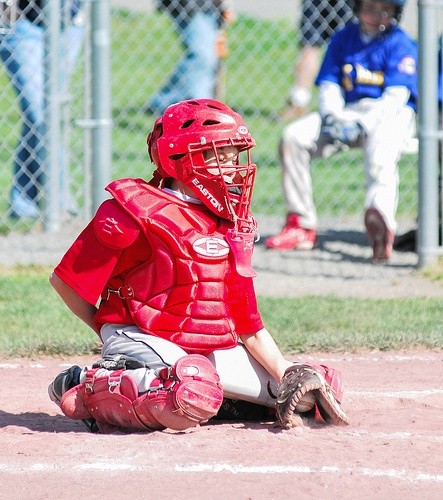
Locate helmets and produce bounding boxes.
[146,99,255,220]
[354,0,405,23]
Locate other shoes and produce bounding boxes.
[48,365,98,433]
[271,102,305,124]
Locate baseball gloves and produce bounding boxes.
[271,358,351,428]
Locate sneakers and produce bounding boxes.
[363,207,395,265]
[264,213,316,251]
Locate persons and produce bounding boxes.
[48,98,341,432]
[0,0,89,222]
[143,1,227,119]
[267,0,443,258]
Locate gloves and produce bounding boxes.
[321,114,344,142]
[339,121,368,148]
